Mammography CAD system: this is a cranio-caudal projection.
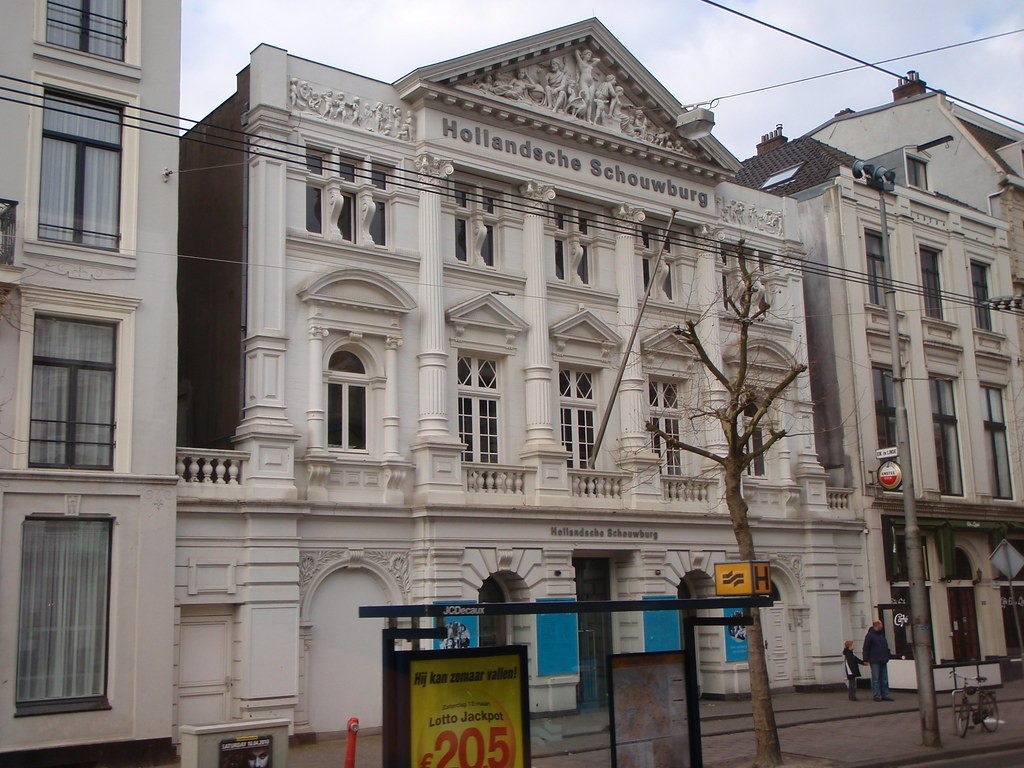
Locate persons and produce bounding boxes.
[247,748,268,768]
[863,620,894,702]
[843,641,868,701]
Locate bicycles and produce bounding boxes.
[947,670,1000,739]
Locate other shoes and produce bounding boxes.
[873,698,882,702]
[882,698,895,702]
[849,698,859,701]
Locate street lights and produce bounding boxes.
[850,160,946,751]
